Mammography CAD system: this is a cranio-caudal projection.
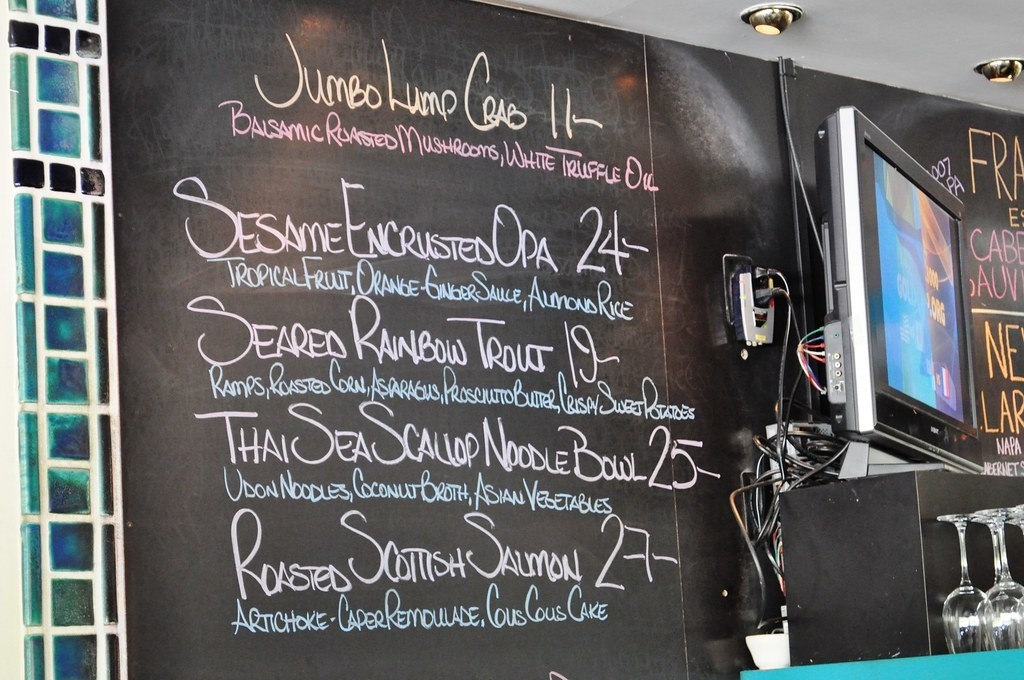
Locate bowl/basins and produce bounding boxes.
[745,634,791,670]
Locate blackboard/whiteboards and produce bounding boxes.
[102,0,1024,680]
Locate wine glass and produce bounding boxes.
[970,508,1024,650]
[936,513,996,654]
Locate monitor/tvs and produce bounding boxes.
[816,104,985,476]
